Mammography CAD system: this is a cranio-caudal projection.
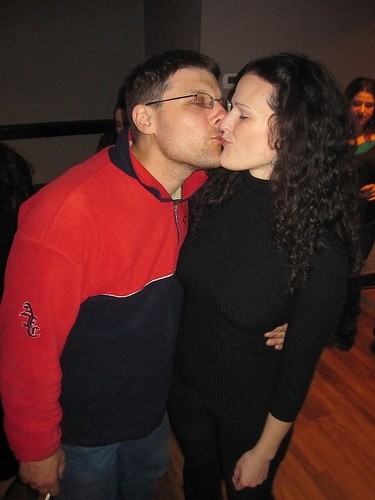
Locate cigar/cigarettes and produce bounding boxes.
[44,492,52,500]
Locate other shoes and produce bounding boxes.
[337,314,356,351]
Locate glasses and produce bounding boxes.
[145,92,232,114]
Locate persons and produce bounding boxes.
[326,76,375,351]
[0,50,288,500]
[95,83,132,154]
[167,52,362,500]
